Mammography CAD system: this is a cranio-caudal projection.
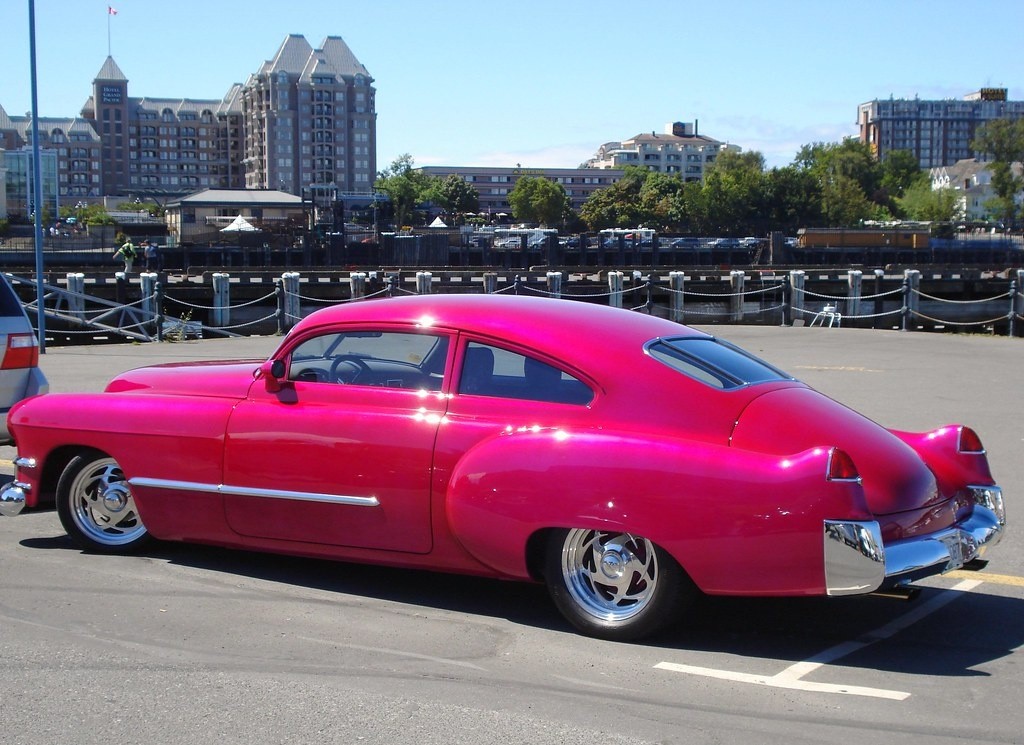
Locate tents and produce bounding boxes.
[219,214,263,233]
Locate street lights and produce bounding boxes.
[134,198,140,223]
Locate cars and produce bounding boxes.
[1,273,49,446]
[668,238,701,248]
[636,240,663,248]
[469,239,478,246]
[603,239,633,247]
[558,237,568,247]
[568,238,592,248]
[530,236,549,248]
[784,236,799,245]
[707,237,767,248]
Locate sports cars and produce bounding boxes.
[4,292,1006,642]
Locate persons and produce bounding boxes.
[112,237,137,273]
[143,240,159,273]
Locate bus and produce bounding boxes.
[600,227,656,243]
[494,228,559,249]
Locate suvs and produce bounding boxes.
[498,240,520,249]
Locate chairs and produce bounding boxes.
[523,357,561,385]
[459,348,494,394]
